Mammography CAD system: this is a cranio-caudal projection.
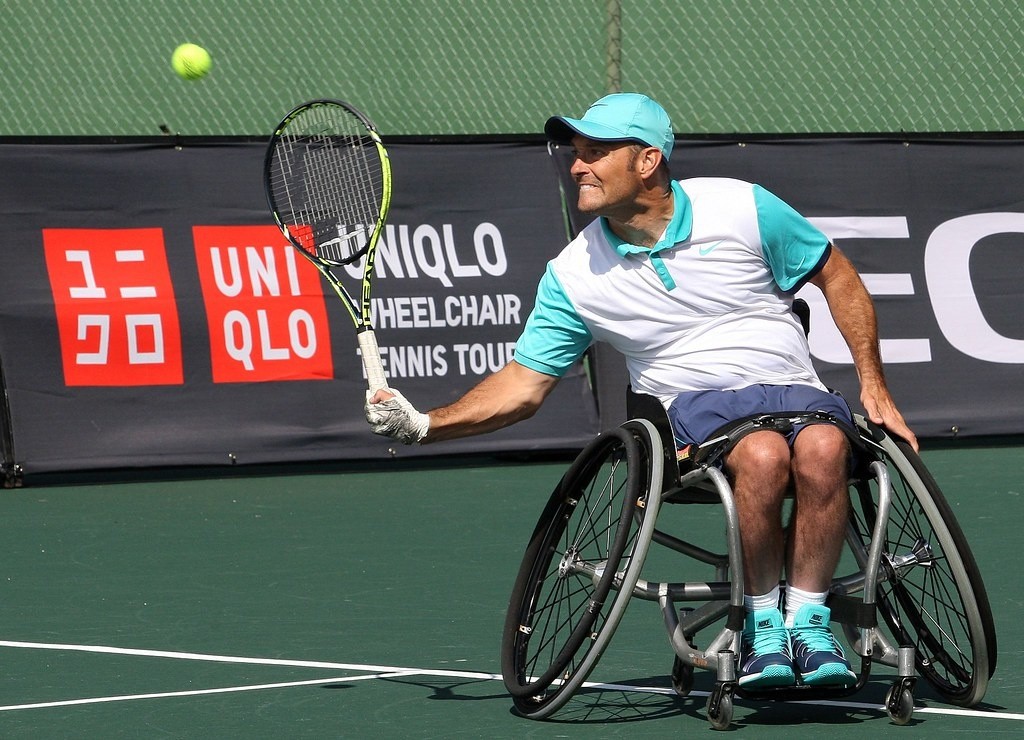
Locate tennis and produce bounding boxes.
[172,44,211,77]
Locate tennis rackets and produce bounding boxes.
[263,98,393,406]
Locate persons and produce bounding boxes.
[356,91,920,687]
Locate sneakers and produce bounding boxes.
[738,608,799,689]
[783,602,856,684]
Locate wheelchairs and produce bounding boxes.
[501,300,1000,732]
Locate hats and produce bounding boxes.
[545,93,674,161]
